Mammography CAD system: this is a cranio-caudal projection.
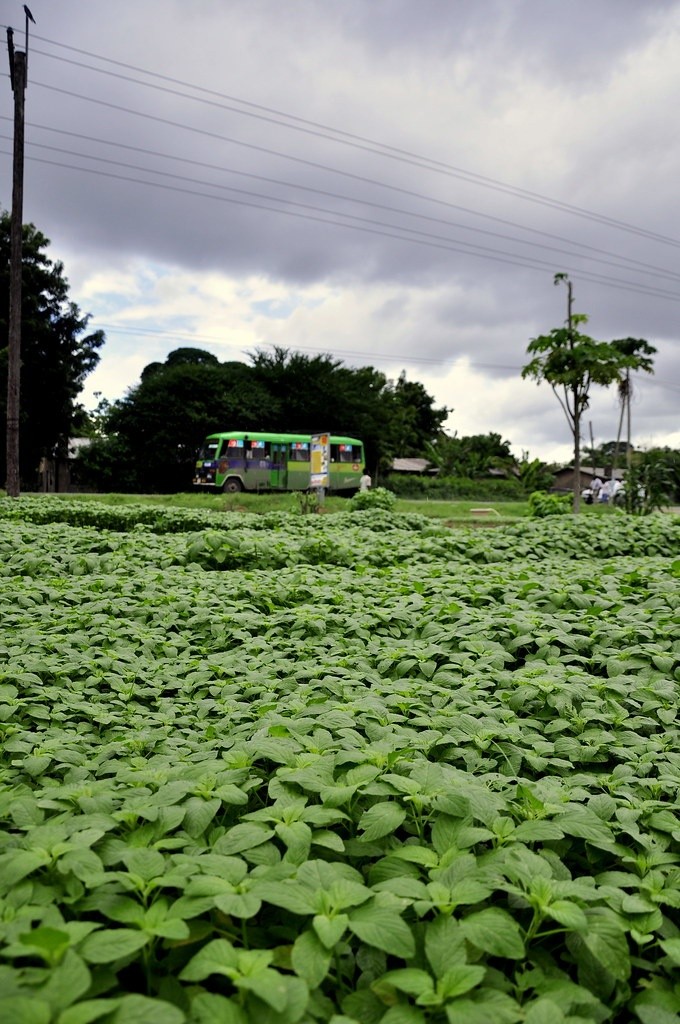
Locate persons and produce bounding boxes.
[360,469,371,491]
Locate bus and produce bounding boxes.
[193,431,366,497]
